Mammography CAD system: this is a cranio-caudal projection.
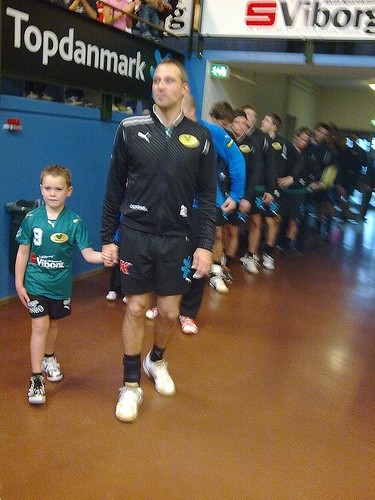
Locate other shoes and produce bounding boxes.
[278,237,302,258]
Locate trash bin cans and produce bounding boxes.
[6,199,41,272]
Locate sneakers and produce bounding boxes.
[115,382,143,423]
[240,252,260,274]
[27,375,46,404]
[123,296,128,303]
[262,253,275,270]
[142,348,175,396]
[220,266,234,285]
[209,276,229,294]
[179,315,198,335]
[210,264,227,279]
[145,305,158,320]
[42,354,63,382]
[105,290,119,301]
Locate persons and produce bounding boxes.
[106,93,375,336]
[102,59,216,422]
[15,165,112,405]
[22,0,172,115]
